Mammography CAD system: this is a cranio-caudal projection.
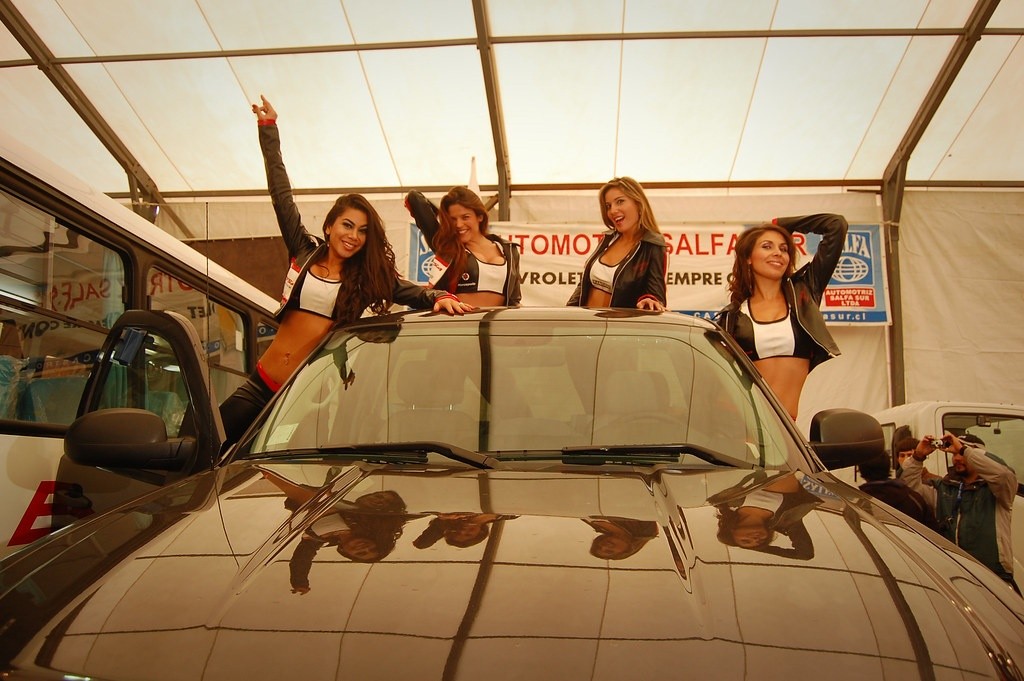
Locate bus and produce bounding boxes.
[0,137,282,578]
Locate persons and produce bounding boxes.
[581,513,659,561]
[711,212,848,424]
[404,186,523,306]
[261,471,410,596]
[412,510,521,548]
[858,452,939,532]
[895,438,942,486]
[220,93,475,460]
[565,176,668,311]
[706,470,825,560]
[899,432,1018,584]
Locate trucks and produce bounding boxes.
[844,400,1024,589]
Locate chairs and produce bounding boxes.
[582,368,705,448]
[377,357,478,451]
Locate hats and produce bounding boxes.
[956,434,986,452]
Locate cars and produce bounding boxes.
[1,308,1024,681]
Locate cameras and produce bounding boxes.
[931,438,945,450]
